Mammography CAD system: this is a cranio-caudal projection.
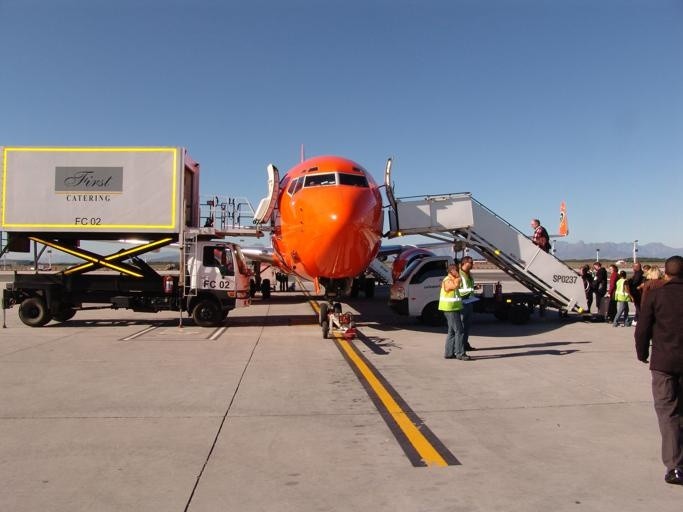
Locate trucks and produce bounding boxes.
[388,256,544,327]
[2,241,251,327]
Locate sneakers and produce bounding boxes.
[464,343,475,351]
[662,468,682,487]
[444,353,470,362]
[598,313,637,328]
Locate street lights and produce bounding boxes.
[552,239,556,255]
[47,247,51,268]
[633,239,638,263]
[596,248,600,262]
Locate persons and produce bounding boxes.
[529,218,548,253]
[579,261,664,328]
[457,256,480,351]
[436,264,470,361]
[633,255,682,484]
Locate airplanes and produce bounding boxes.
[117,155,570,339]
